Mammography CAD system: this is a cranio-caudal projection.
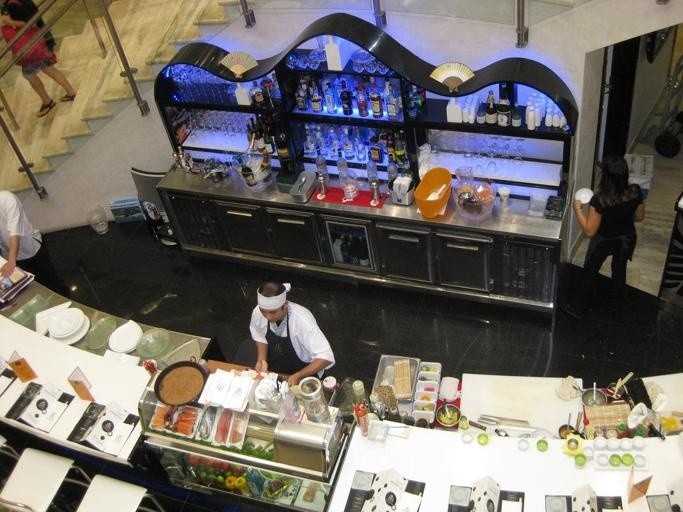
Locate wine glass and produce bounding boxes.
[428,130,527,170]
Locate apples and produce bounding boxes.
[186,454,247,491]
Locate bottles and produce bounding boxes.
[260,150,272,183]
[236,155,258,187]
[173,146,195,172]
[477,82,566,131]
[340,234,370,266]
[583,418,594,440]
[389,406,401,423]
[246,72,289,156]
[294,71,423,122]
[304,123,409,201]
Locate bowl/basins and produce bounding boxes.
[558,424,574,440]
[435,404,461,427]
[581,388,607,408]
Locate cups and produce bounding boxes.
[518,438,549,452]
[565,436,646,468]
[353,410,369,437]
[498,187,511,207]
[189,110,254,140]
[462,93,479,124]
[462,432,489,445]
[352,57,390,75]
[286,55,321,70]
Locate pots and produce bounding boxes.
[153,360,208,407]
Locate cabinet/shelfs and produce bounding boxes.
[153,13,579,334]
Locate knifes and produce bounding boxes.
[469,420,490,432]
[480,413,530,428]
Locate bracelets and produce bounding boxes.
[575,206,583,211]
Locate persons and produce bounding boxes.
[249,282,337,386]
[0,190,67,297]
[0,0,76,117]
[559,155,645,320]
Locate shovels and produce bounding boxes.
[426,184,447,201]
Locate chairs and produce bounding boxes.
[0,434,166,512]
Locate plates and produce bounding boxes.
[35,301,73,336]
[136,327,171,358]
[294,479,332,511]
[108,319,144,354]
[48,308,90,346]
[8,294,51,329]
[85,315,117,350]
[148,399,250,451]
[574,187,594,205]
